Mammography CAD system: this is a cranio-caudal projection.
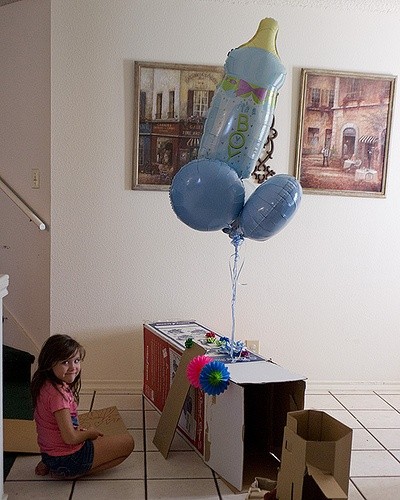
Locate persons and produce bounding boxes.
[30,333,134,480]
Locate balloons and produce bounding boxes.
[197,18,287,179]
[169,159,246,232]
[237,174,303,242]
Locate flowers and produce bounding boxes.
[187,355,230,395]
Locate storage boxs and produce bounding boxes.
[144,319,308,493]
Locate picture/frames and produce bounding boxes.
[131,60,223,191]
[295,68,397,199]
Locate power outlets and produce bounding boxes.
[246,339,259,354]
[32,168,39,189]
